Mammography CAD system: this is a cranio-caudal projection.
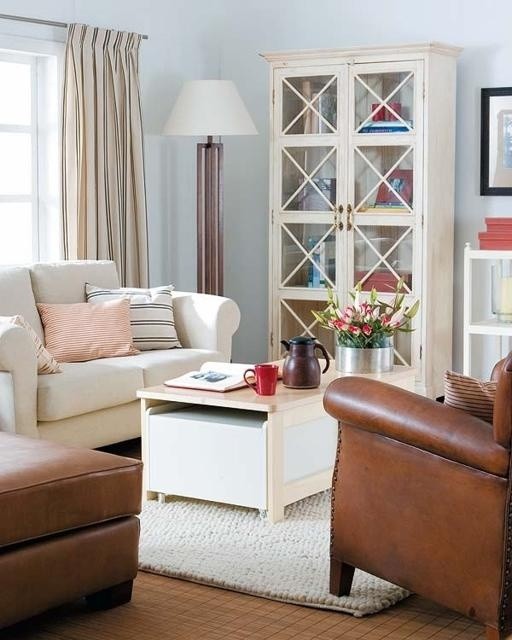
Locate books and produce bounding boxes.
[295,177,337,211]
[369,168,414,208]
[358,102,413,133]
[306,235,333,289]
[163,359,283,394]
[354,270,413,280]
[311,92,337,134]
[477,217,511,251]
[300,78,313,133]
[353,280,412,294]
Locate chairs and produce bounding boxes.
[320,345,511,639]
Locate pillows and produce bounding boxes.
[441,358,500,422]
[0,278,185,380]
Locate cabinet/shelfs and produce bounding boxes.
[256,42,462,400]
[463,241,512,380]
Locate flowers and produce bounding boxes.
[308,274,421,349]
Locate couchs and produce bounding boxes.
[0,260,243,451]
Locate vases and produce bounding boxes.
[334,341,397,376]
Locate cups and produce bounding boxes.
[244,362,279,397]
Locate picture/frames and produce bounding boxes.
[480,86,512,197]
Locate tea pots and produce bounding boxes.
[282,336,332,389]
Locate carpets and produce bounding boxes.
[122,440,414,617]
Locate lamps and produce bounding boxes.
[161,75,259,297]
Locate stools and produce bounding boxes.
[0,424,144,631]
